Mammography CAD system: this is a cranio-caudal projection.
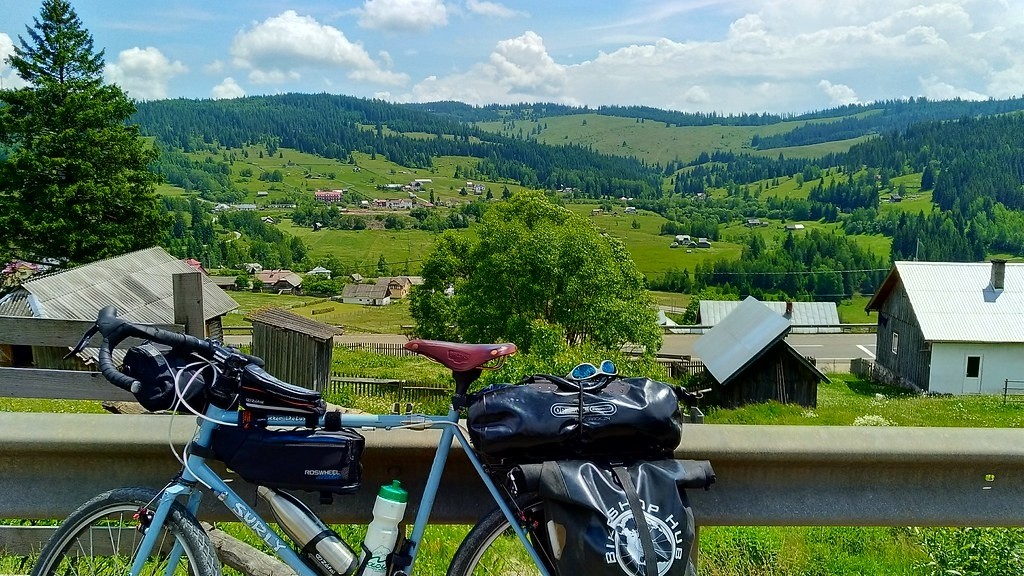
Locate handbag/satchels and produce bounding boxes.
[240,424,367,494]
[467,374,683,455]
[505,457,698,576]
[240,363,328,418]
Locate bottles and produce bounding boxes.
[361,480,409,576]
[256,485,358,576]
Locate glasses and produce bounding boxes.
[562,359,619,381]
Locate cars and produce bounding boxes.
[624,209,630,213]
[670,242,678,248]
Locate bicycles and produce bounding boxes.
[30,307,548,576]
